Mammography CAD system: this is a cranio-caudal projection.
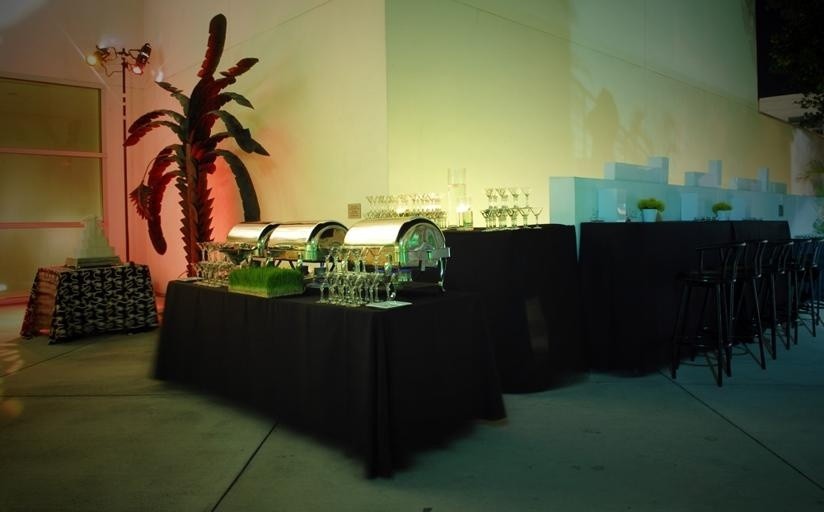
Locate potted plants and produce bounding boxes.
[638,197,665,223]
[712,201,732,221]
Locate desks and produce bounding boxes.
[31,265,156,343]
[157,279,486,482]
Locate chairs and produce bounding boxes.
[670,235,824,390]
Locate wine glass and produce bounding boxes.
[313,244,396,308]
[367,195,449,232]
[480,184,544,232]
[191,241,239,288]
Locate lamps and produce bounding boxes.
[86,42,152,264]
